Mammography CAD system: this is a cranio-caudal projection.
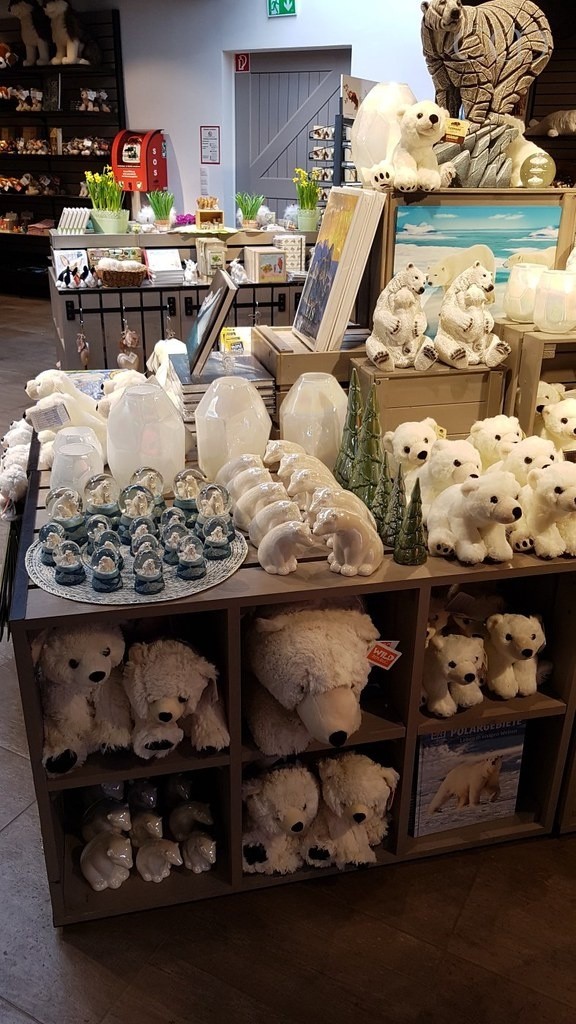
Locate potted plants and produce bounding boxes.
[234,191,265,228]
[146,189,174,231]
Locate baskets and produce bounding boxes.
[96,265,147,287]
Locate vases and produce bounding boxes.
[90,208,129,233]
[297,209,321,232]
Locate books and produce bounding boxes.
[51,265,276,447]
[292,185,386,351]
[195,234,307,284]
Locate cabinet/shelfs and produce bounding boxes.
[0,9,127,302]
[11,324,575,928]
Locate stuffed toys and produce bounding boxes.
[8,1,89,65]
[0,84,115,196]
[31,622,230,776]
[243,595,398,874]
[0,339,187,522]
[364,259,513,370]
[371,102,456,192]
[384,381,576,564]
[425,595,545,716]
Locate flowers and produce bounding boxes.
[293,168,324,208]
[84,164,125,214]
